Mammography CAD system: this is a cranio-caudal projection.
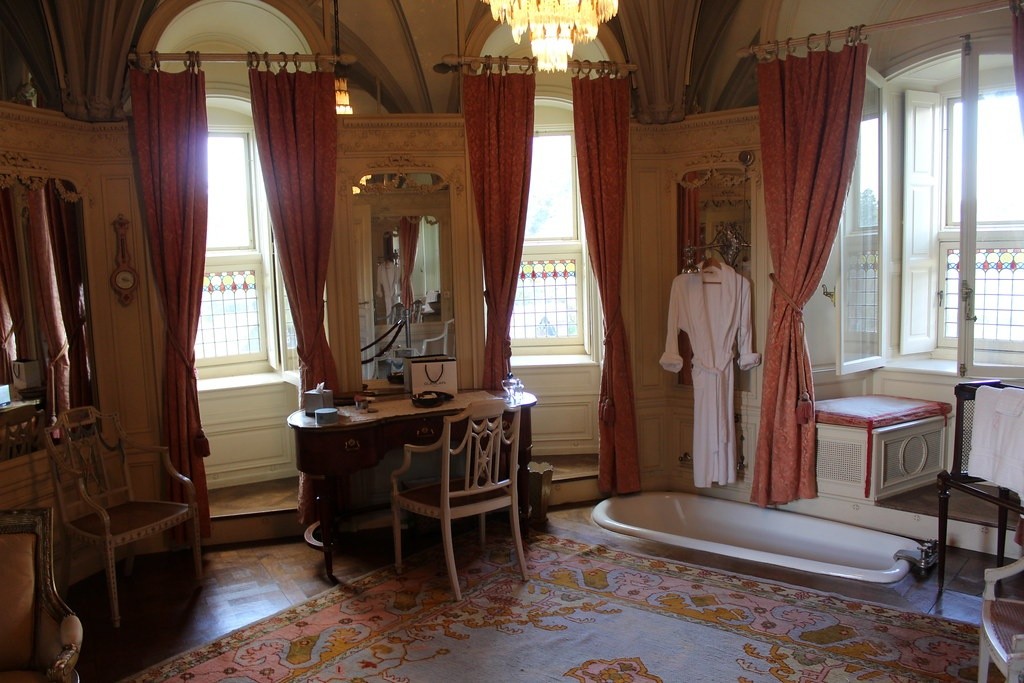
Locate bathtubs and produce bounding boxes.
[590,489,920,584]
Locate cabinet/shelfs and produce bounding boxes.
[936,380,1024,590]
[286,388,538,577]
[814,394,954,500]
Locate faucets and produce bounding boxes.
[893,538,940,582]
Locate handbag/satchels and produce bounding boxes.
[410,359,458,395]
[404,354,449,392]
[11,360,41,388]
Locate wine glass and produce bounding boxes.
[501,381,516,402]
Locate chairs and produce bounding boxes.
[0,405,46,463]
[391,396,529,601]
[0,505,83,683]
[42,406,202,631]
[977,555,1024,683]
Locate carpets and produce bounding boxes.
[115,528,1009,683]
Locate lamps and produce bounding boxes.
[681,240,722,274]
[480,0,620,72]
[333,1,353,115]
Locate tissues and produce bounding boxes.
[303,381,334,417]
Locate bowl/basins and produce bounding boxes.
[411,393,445,408]
[387,373,403,383]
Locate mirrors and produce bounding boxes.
[361,213,454,383]
[677,167,753,393]
[0,166,102,464]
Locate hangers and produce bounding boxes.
[700,246,721,285]
